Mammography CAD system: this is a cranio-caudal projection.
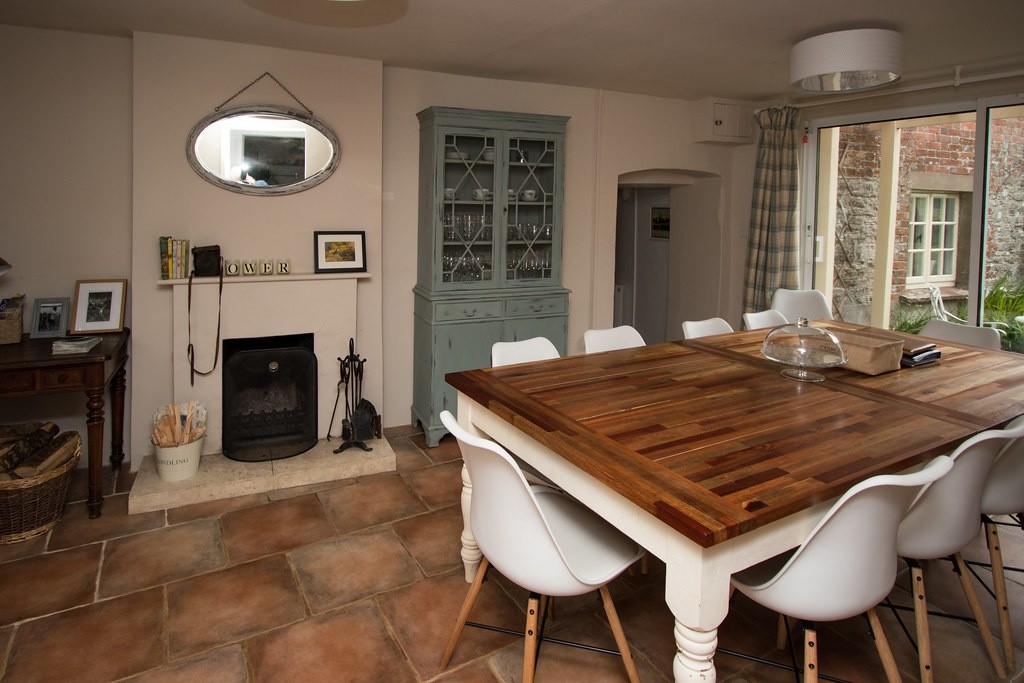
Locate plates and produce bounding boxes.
[521,198,538,202]
[445,197,457,200]
[473,197,492,200]
[446,152,471,160]
[508,198,516,200]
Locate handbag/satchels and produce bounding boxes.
[191,245,222,277]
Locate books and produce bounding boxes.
[160,236,190,280]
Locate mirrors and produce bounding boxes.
[186,105,342,196]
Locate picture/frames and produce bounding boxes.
[70,278,126,334]
[649,206,670,241]
[312,229,366,273]
[30,297,69,339]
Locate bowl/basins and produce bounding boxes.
[482,147,495,160]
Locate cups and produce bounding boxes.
[473,188,489,197]
[444,188,456,197]
[520,190,536,198]
[444,214,552,242]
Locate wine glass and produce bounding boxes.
[443,255,550,281]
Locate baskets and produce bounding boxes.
[0,455,80,546]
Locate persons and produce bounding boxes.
[50,305,58,331]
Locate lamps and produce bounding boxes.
[0,258,11,275]
[788,30,904,92]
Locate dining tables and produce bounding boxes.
[445,316,1024,683]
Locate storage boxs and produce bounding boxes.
[822,327,905,375]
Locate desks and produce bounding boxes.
[0,326,131,518]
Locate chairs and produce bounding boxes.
[681,317,734,340]
[731,417,1024,683]
[744,310,788,330]
[919,319,1000,351]
[440,412,646,683]
[584,325,634,356]
[491,337,560,368]
[770,289,834,325]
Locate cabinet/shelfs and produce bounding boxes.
[689,99,755,144]
[413,105,570,449]
